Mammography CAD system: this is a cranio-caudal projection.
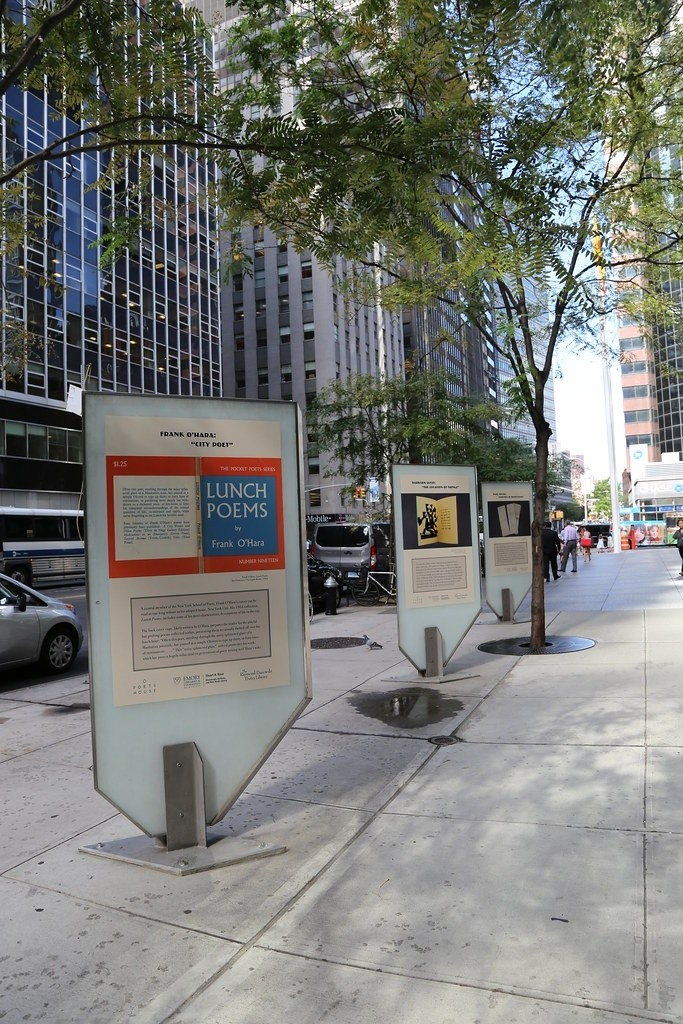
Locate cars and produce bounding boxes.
[0,570,84,672]
[306,541,344,614]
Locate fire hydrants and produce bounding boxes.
[322,575,339,615]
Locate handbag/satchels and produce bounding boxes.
[581,538,592,547]
[559,549,564,555]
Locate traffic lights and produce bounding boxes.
[357,487,361,498]
[361,488,366,499]
[353,488,357,499]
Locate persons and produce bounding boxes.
[597,533,613,553]
[669,520,683,577]
[621,523,660,544]
[542,520,591,582]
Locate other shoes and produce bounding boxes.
[589,559,591,561]
[546,578,550,582]
[679,573,683,576]
[554,575,561,580]
[584,561,586,562]
[558,569,565,572]
[571,570,577,572]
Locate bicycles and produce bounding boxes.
[349,562,397,607]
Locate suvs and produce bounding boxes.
[314,520,390,586]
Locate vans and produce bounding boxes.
[576,523,612,547]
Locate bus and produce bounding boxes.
[618,504,683,547]
[0,505,85,588]
